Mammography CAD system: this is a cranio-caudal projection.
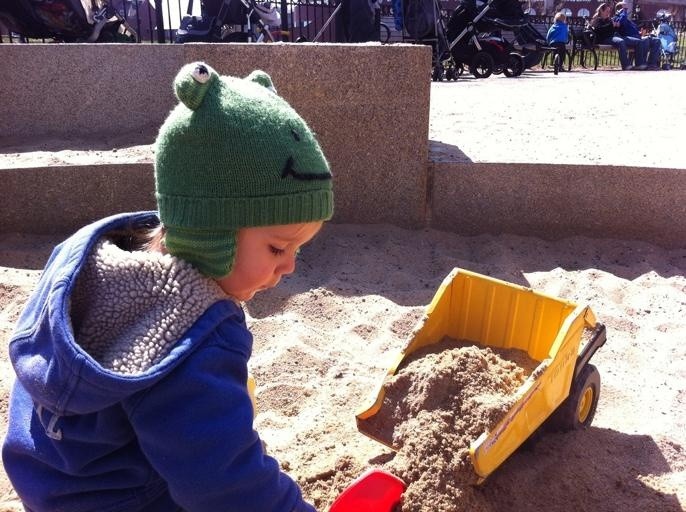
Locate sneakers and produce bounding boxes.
[622,62,662,71]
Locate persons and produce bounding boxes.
[0,61,336,512]
[545,12,570,72]
[588,2,649,70]
[612,1,661,70]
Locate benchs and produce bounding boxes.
[373,6,651,73]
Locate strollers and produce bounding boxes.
[1,0,137,45]
[176,0,282,41]
[442,1,556,83]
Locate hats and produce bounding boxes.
[153,61,335,281]
